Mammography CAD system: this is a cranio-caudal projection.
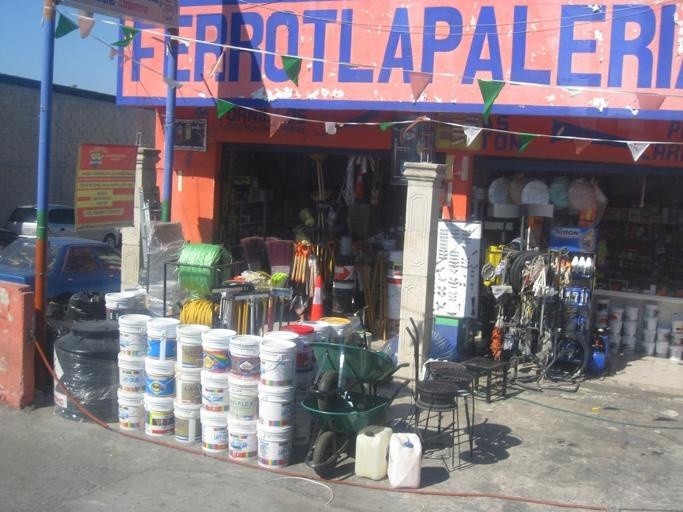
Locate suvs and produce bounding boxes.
[1,204,120,249]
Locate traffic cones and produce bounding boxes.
[311,273,323,320]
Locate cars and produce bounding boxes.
[0,235,122,320]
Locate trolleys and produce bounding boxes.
[300,376,409,480]
[303,304,409,412]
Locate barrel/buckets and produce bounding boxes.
[260,340,296,386]
[624,337,635,353]
[669,333,682,345]
[201,329,236,372]
[671,317,683,334]
[596,299,609,315]
[644,317,659,329]
[610,308,624,320]
[655,341,669,357]
[596,314,607,327]
[623,320,638,336]
[626,306,640,320]
[641,342,654,355]
[174,407,202,443]
[117,389,145,430]
[258,384,295,428]
[321,316,352,344]
[264,331,300,345]
[299,320,330,345]
[201,371,229,414]
[229,335,262,377]
[387,433,422,488]
[202,413,229,453]
[280,324,314,371]
[354,425,393,481]
[656,327,670,340]
[147,318,182,360]
[228,419,258,462]
[608,321,623,334]
[645,302,660,317]
[608,336,622,351]
[642,330,656,342]
[145,396,174,436]
[146,358,176,399]
[230,377,259,422]
[117,354,147,389]
[292,402,311,446]
[105,292,145,321]
[176,324,211,367]
[176,367,203,410]
[119,313,151,356]
[258,425,292,469]
[669,346,683,361]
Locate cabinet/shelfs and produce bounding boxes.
[217,144,301,271]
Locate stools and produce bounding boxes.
[425,361,476,462]
[416,379,465,474]
